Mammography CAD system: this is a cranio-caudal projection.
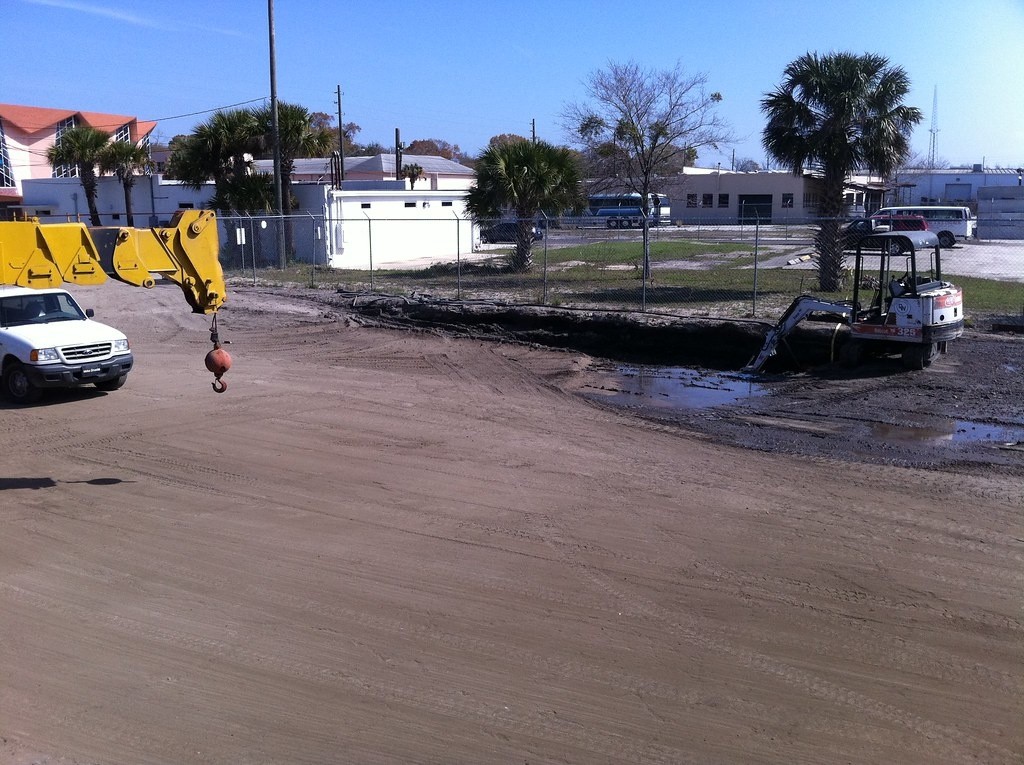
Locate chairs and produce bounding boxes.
[23,303,46,319]
[889,281,905,298]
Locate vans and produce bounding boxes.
[869,214,929,232]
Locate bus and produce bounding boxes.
[873,204,974,248]
[541,193,670,228]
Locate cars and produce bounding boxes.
[971,212,977,237]
[0,280,134,406]
[480,222,543,243]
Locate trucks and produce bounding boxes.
[813,218,936,255]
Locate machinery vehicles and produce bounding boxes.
[743,229,965,377]
[0,206,232,394]
